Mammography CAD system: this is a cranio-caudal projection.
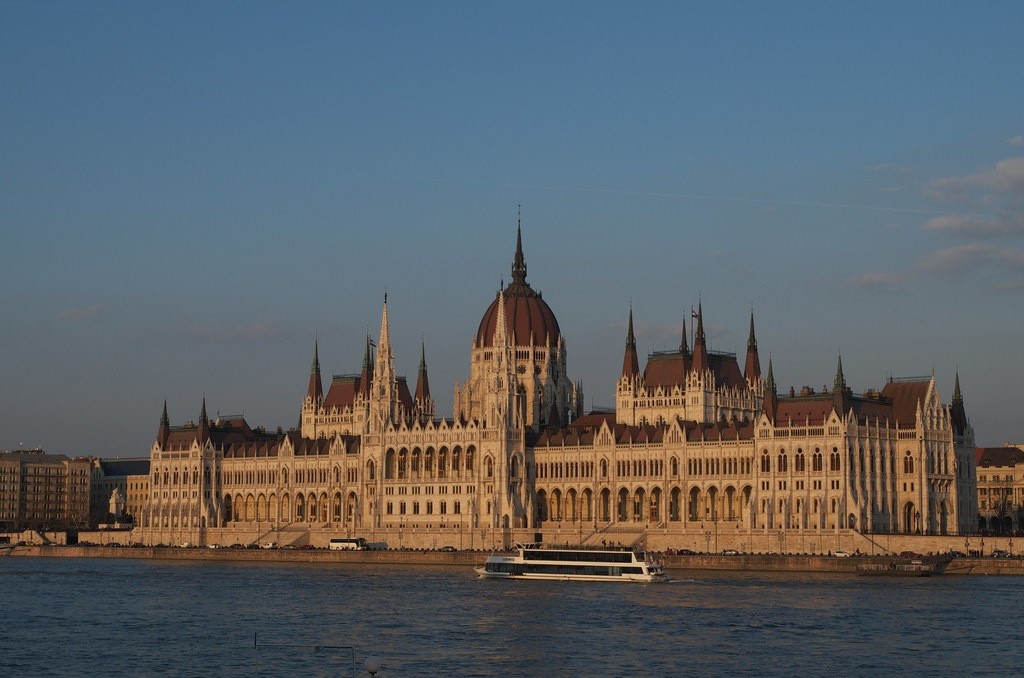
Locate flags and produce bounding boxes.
[692,311,700,320]
[370,337,377,347]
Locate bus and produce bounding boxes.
[330,537,366,551]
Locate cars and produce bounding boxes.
[441,546,455,552]
[210,543,297,549]
[992,551,1010,558]
[721,550,739,556]
[900,551,918,557]
[833,550,849,557]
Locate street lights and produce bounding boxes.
[344,529,352,551]
[481,531,486,551]
[399,530,403,551]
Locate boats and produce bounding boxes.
[473,549,665,582]
